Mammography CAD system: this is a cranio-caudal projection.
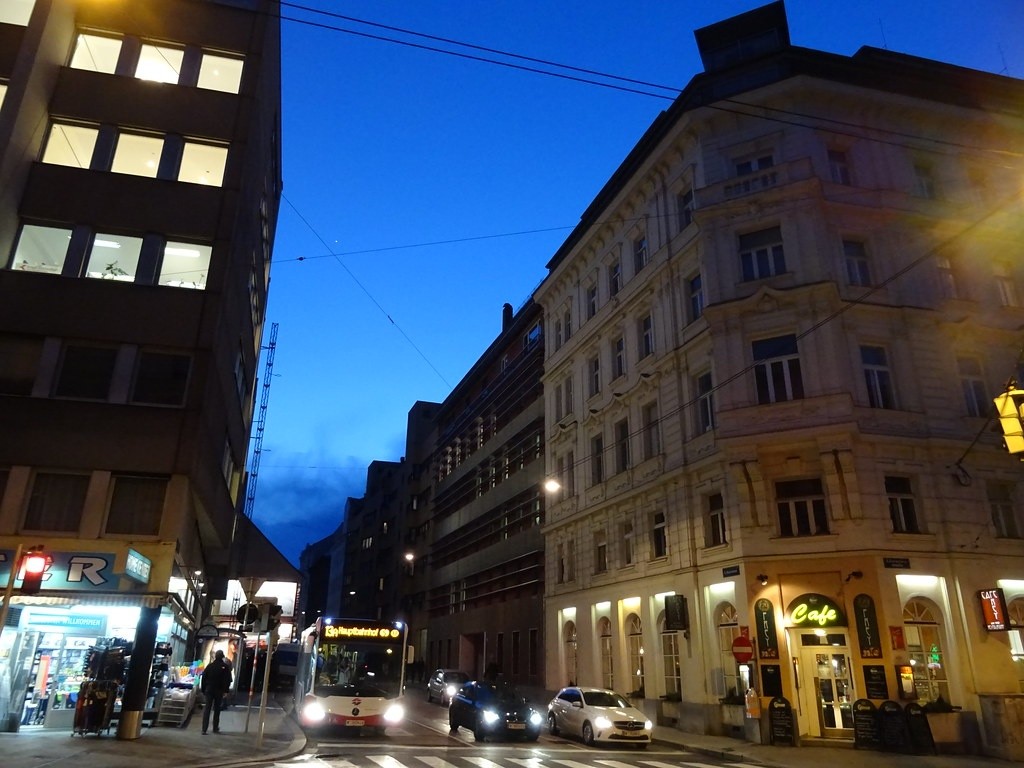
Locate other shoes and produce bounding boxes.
[202,731,206,735]
[214,729,219,731]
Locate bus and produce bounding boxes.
[290,616,409,736]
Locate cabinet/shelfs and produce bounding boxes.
[155,690,193,728]
[24,647,87,699]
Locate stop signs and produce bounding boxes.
[732,637,753,663]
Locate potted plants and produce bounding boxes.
[661,692,681,720]
[627,692,643,714]
[720,696,745,726]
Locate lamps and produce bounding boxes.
[175,565,207,596]
[756,574,769,586]
[845,571,863,582]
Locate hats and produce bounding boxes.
[216,651,224,658]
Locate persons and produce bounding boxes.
[320,653,353,685]
[418,657,425,682]
[200,650,232,736]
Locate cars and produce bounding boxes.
[548,686,655,749]
[447,677,544,742]
[425,668,474,706]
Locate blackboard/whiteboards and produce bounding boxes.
[852,699,883,747]
[768,696,794,742]
[878,700,907,753]
[904,703,938,755]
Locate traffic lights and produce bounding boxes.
[17,543,49,594]
[258,603,284,636]
